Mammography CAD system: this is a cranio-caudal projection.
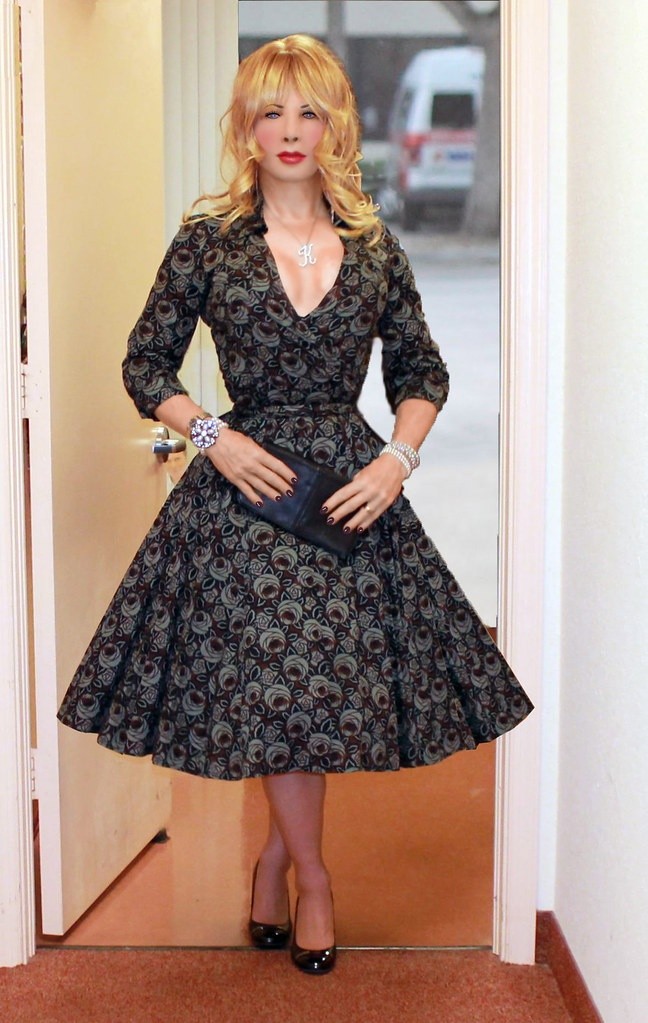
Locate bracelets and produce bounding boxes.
[378,442,421,479]
[186,412,229,457]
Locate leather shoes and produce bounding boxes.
[290,890,336,973]
[249,859,292,949]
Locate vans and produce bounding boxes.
[391,45,485,230]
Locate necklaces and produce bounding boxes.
[265,203,323,267]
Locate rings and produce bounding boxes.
[365,505,373,515]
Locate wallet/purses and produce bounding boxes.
[232,441,361,559]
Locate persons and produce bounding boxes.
[59,36,535,973]
